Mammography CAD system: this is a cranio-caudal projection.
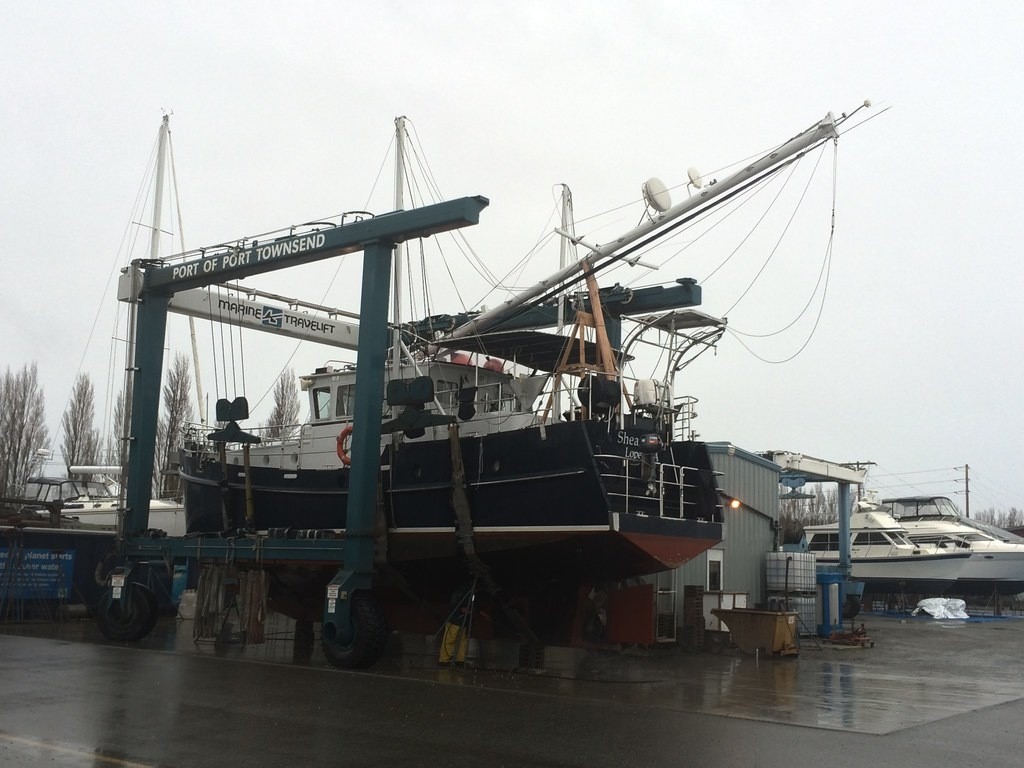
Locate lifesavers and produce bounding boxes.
[336,425,354,466]
[96,582,157,643]
[320,592,388,671]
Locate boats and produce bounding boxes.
[27,461,187,537]
[862,492,1024,596]
[802,495,975,563]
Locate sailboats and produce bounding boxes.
[177,97,894,634]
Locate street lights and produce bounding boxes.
[36,449,51,493]
